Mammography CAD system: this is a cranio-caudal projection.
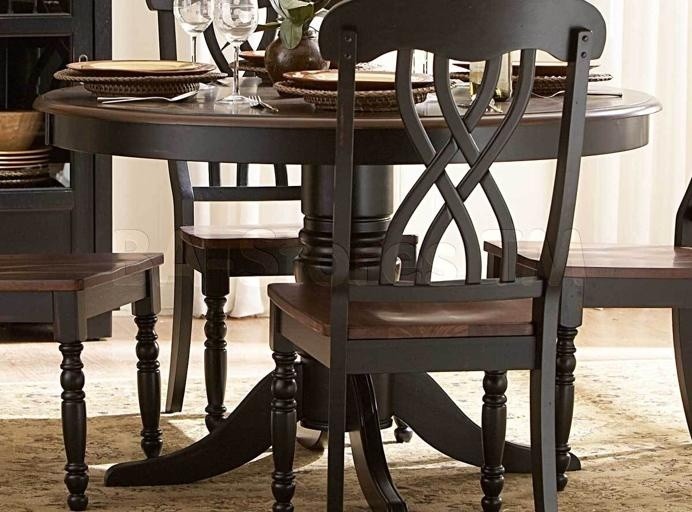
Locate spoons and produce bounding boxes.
[102,90,198,105]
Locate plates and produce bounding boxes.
[279,69,433,91]
[450,62,600,77]
[238,50,266,66]
[65,59,217,77]
[0,144,53,171]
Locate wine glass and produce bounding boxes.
[173,0,258,107]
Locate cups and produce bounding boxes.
[452,82,475,109]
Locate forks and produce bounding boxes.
[247,95,280,114]
